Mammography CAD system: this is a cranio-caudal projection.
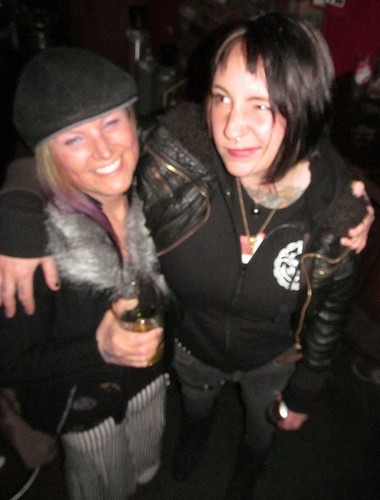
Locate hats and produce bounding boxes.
[14,48,137,148]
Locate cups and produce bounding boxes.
[104,278,164,367]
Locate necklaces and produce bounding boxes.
[236,145,300,249]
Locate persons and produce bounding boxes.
[0,8,375,500]
[0,40,375,500]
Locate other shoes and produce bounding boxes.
[226,462,265,499]
[172,436,203,483]
